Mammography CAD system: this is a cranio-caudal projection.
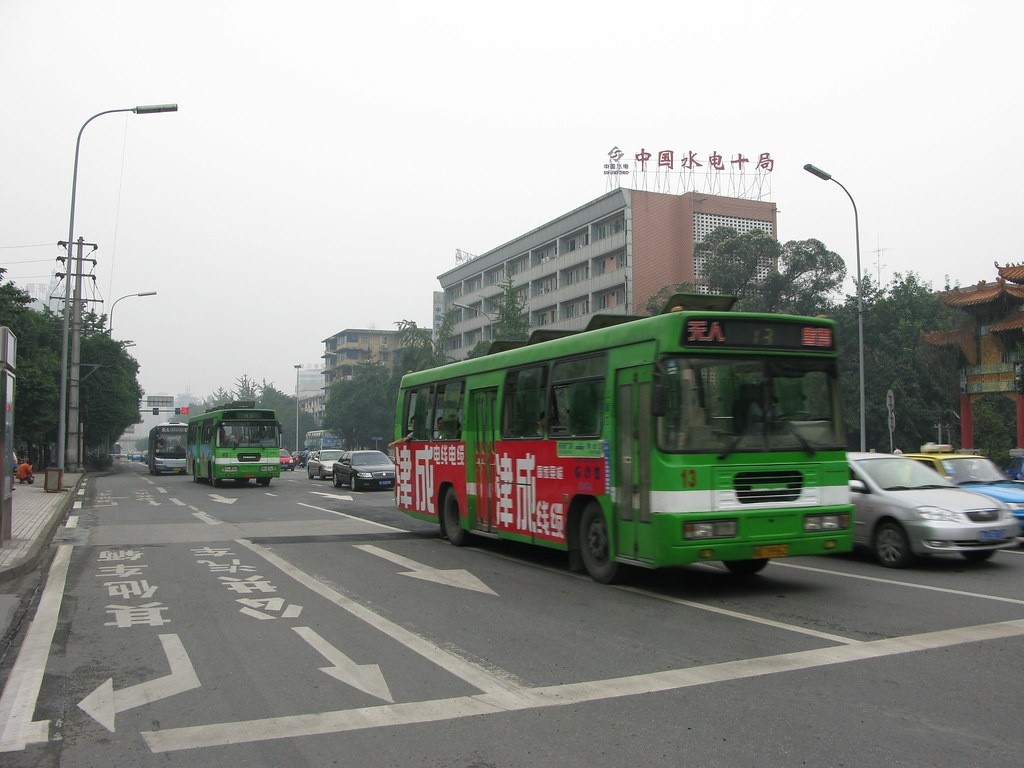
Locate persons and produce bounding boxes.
[12,446,35,491]
[300,448,309,467]
[894,446,903,454]
[745,382,784,436]
[388,415,460,448]
[535,411,552,436]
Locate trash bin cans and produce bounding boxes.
[44,467,62,491]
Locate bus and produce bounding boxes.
[304,430,340,450]
[147,422,189,476]
[393,291,857,587]
[185,401,283,488]
[108,442,122,460]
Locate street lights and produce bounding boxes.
[802,162,866,453]
[109,291,158,342]
[452,300,494,344]
[323,350,354,380]
[293,364,302,452]
[56,103,178,481]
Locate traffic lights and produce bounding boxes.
[182,407,189,414]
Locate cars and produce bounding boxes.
[121,453,127,457]
[307,449,345,480]
[838,452,1021,569]
[890,442,1024,535]
[290,449,318,468]
[127,450,148,464]
[279,449,295,471]
[332,450,396,492]
